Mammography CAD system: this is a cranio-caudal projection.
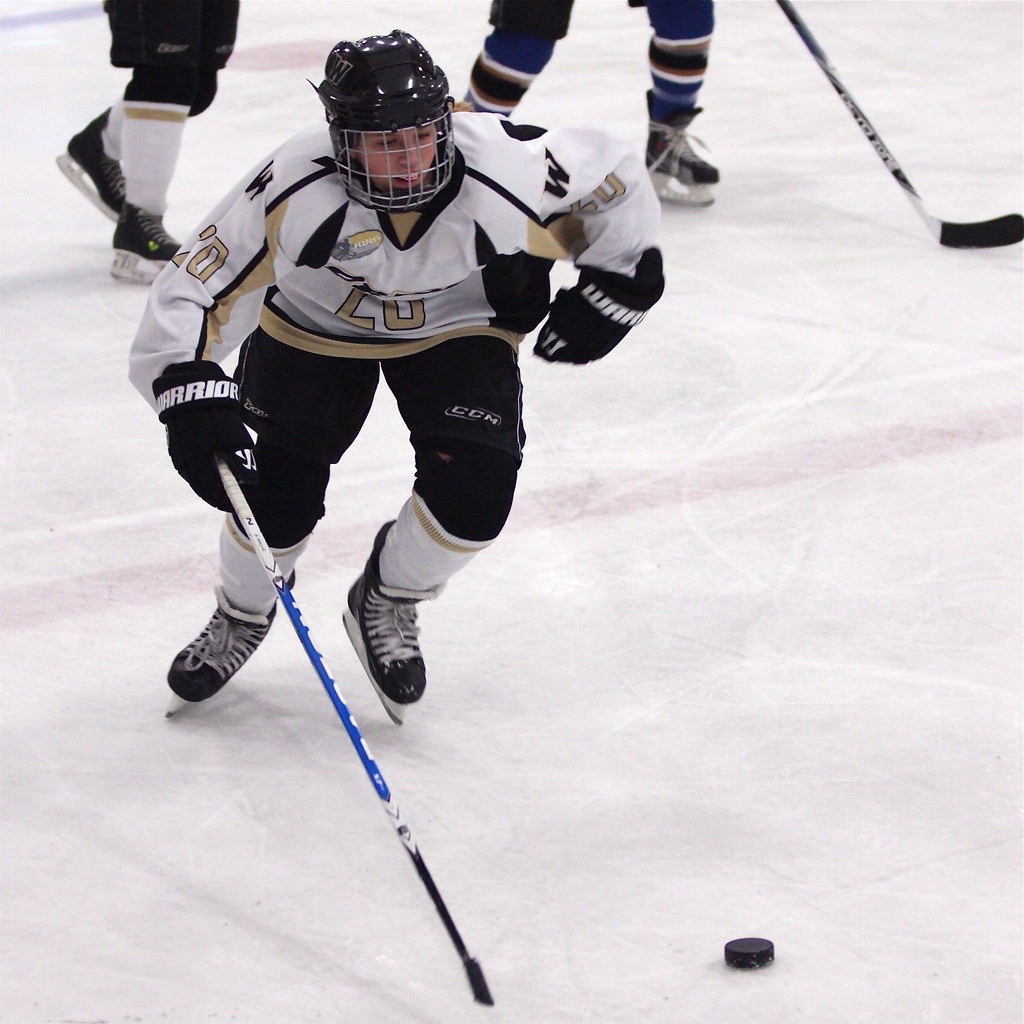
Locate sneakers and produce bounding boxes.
[168,586,276,713]
[110,204,181,283]
[55,108,125,212]
[341,519,446,728]
[645,127,720,206]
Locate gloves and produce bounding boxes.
[152,361,276,518]
[534,248,666,363]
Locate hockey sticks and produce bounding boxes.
[775,1,1024,251]
[213,447,497,1008]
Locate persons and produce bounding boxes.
[64,0,244,260]
[125,26,667,707]
[451,0,729,190]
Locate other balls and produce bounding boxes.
[723,936,778,971]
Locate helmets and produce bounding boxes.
[318,29,449,151]
